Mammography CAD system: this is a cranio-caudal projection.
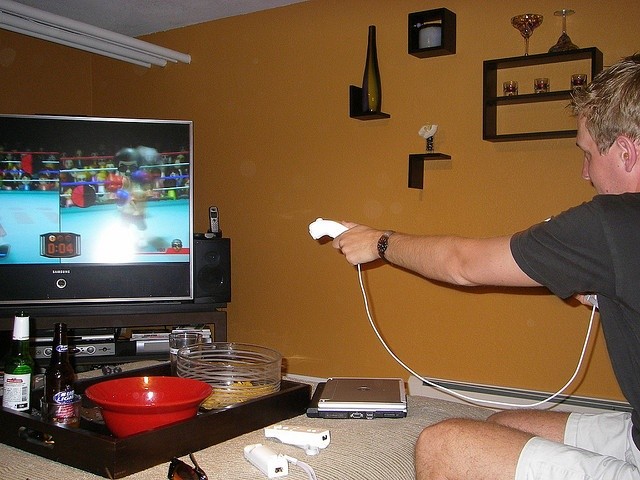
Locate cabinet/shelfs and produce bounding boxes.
[482,46,603,142]
[408,6,456,59]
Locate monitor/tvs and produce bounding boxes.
[1,113,194,306]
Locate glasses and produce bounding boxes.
[168,451,208,480]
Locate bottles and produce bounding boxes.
[3,309,33,414]
[44,323,76,430]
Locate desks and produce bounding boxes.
[0,311,228,363]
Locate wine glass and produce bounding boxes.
[548,9,580,52]
[511,14,544,56]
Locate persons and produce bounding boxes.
[331,51,640,480]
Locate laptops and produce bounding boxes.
[307,376,407,419]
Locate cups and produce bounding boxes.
[169,331,202,377]
[503,81,518,96]
[40,393,82,428]
[570,74,587,89]
[534,78,551,94]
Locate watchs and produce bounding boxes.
[376,230,395,262]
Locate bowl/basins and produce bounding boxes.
[84,374,212,439]
[177,345,283,412]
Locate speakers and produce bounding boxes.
[181,238,230,304]
